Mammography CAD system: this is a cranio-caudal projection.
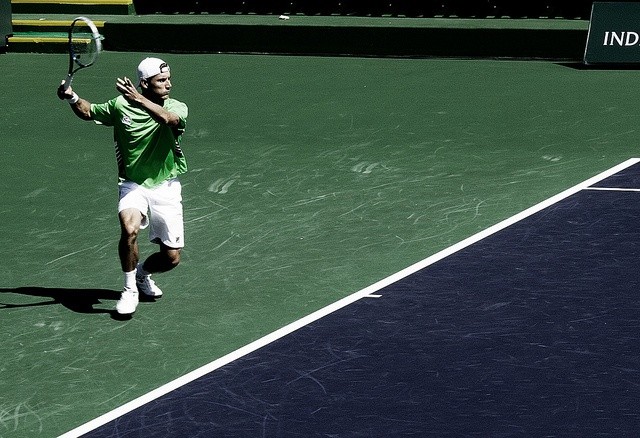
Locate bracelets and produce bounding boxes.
[67,92,80,105]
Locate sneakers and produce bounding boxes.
[116,287,139,314]
[135,274,163,297]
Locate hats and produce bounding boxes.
[135,57,170,89]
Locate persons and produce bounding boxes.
[59,55,191,322]
